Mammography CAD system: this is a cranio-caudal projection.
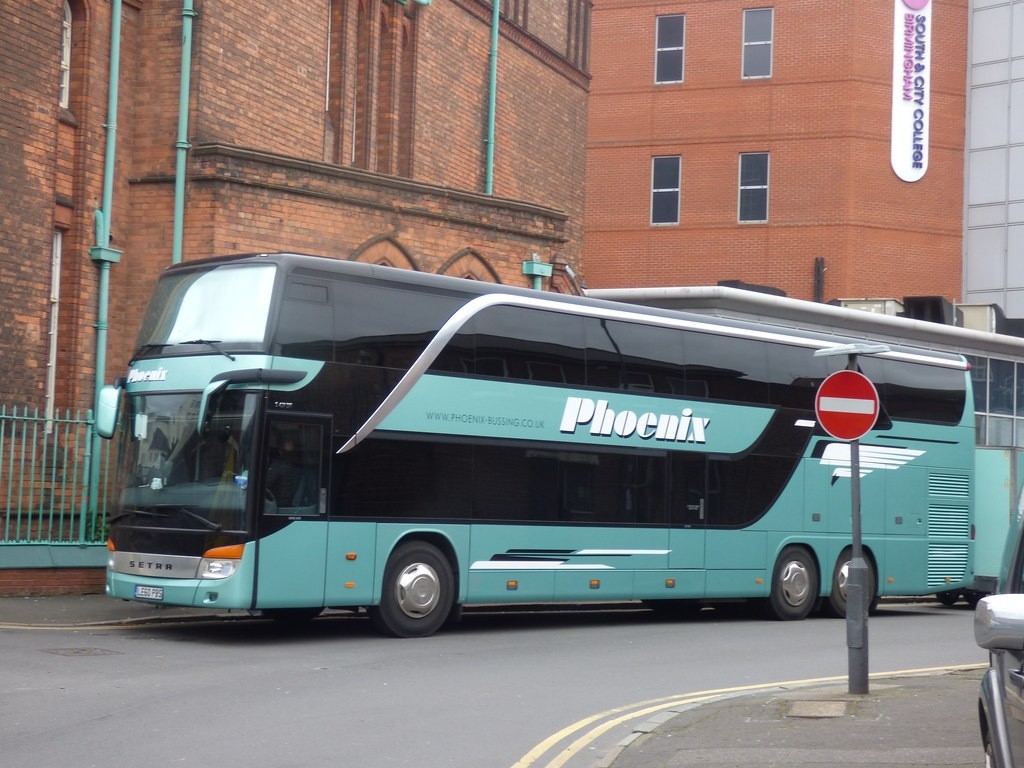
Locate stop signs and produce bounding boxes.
[814,369,880,442]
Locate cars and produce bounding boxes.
[973,483,1024,768]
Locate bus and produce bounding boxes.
[95,252,1024,639]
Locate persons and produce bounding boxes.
[267,434,302,508]
